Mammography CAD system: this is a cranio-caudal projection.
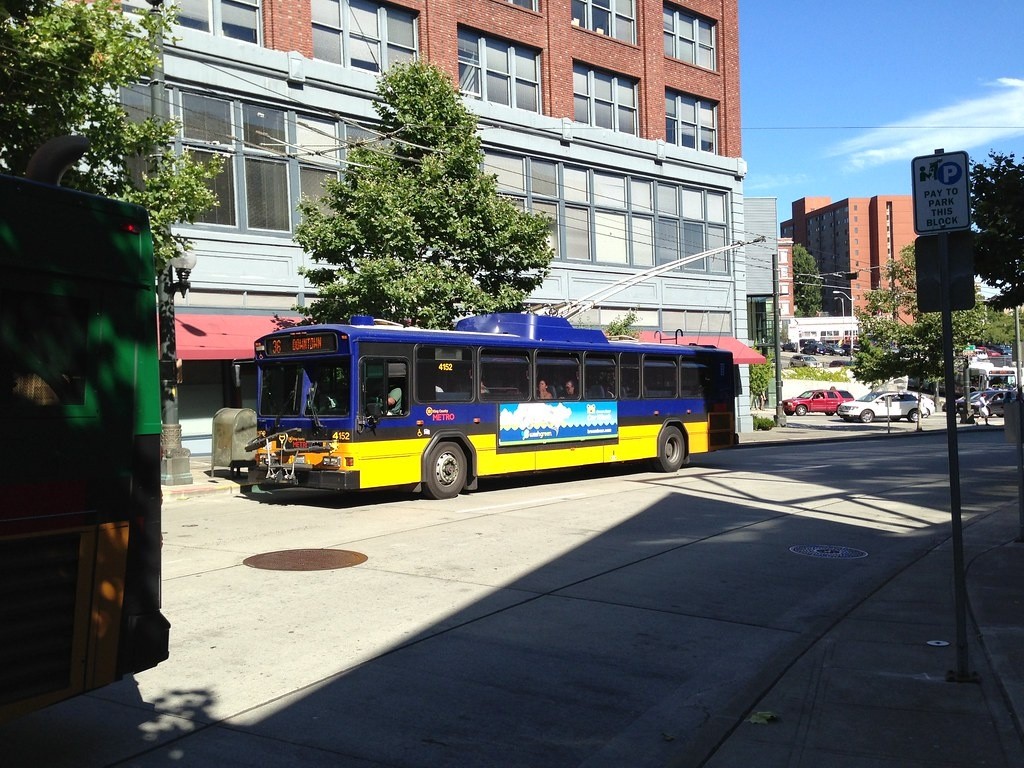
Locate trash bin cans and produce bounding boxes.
[210,408,257,481]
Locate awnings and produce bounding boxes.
[600,328,767,366]
[157,313,317,363]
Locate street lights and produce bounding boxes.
[135,2,194,483]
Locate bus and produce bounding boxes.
[228,310,744,500]
[1,129,179,720]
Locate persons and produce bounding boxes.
[975,394,992,426]
[996,395,1001,400]
[375,378,402,417]
[818,394,823,399]
[558,380,578,400]
[537,379,553,399]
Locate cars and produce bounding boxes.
[778,337,1018,422]
